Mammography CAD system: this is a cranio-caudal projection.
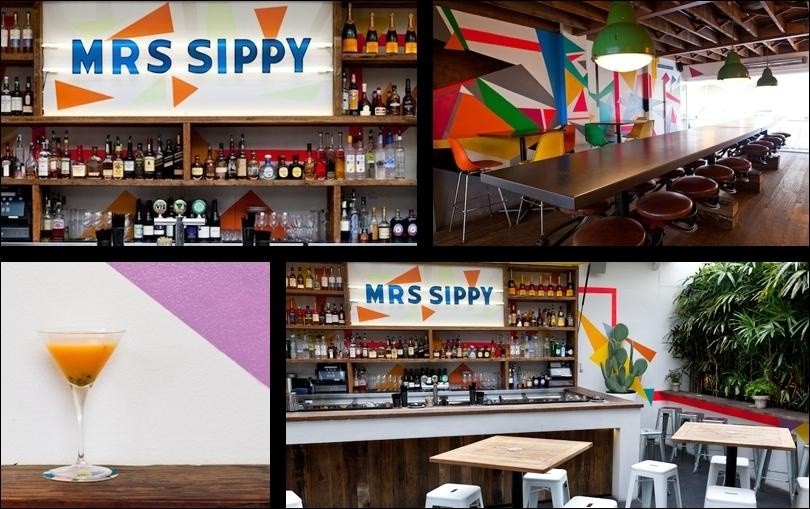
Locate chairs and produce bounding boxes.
[447,117,654,244]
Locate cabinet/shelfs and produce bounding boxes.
[2,0,430,248]
[283,262,579,396]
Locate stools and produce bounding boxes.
[559,132,793,245]
[657,406,810,502]
[704,486,756,508]
[626,459,684,508]
[708,454,751,487]
[793,478,810,508]
[563,494,618,508]
[524,468,570,509]
[285,490,303,508]
[640,431,666,461]
[425,483,484,509]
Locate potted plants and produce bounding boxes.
[743,377,777,409]
[665,368,683,392]
[600,324,648,403]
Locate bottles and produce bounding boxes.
[343,70,416,116]
[404,14,419,55]
[1,12,10,52]
[277,155,289,179]
[205,144,215,180]
[2,144,13,177]
[54,202,64,241]
[289,392,313,411]
[217,143,228,179]
[305,128,406,178]
[229,135,236,178]
[237,133,248,178]
[191,155,203,180]
[2,77,11,114]
[23,77,33,114]
[79,210,131,244]
[249,148,260,178]
[386,13,399,53]
[133,197,221,244]
[40,195,52,241]
[11,12,21,53]
[261,153,275,179]
[290,155,303,179]
[24,12,34,52]
[342,3,358,53]
[15,130,183,179]
[12,77,21,115]
[365,13,379,54]
[285,264,575,390]
[340,188,418,242]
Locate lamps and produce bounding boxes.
[717,2,754,80]
[590,2,657,73]
[757,41,780,86]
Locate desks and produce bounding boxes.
[2,465,270,508]
[672,421,797,486]
[431,434,593,509]
[480,127,763,246]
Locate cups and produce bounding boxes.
[392,394,401,408]
[476,392,484,404]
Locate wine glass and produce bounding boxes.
[258,211,320,242]
[39,329,125,482]
